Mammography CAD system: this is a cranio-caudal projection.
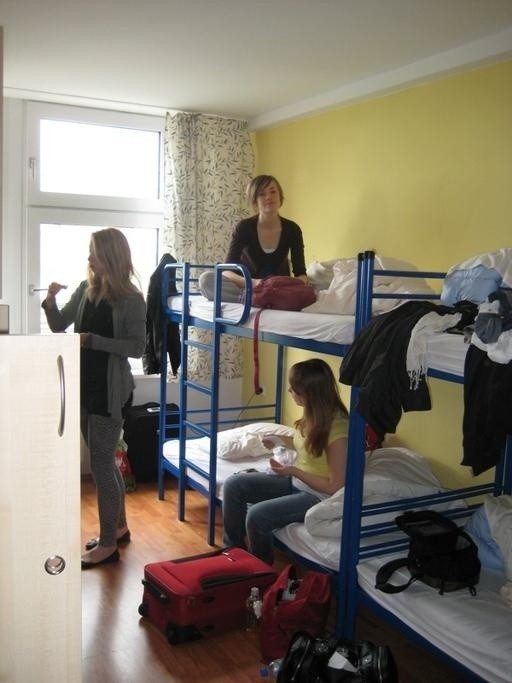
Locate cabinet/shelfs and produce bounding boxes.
[0,333,83,683]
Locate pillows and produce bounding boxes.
[197,421,295,460]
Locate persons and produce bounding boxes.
[40,227,147,569]
[220,358,349,566]
[198,174,308,308]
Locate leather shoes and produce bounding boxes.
[82,548,120,569]
[87,530,130,549]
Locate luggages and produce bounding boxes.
[123,402,181,482]
[138,547,278,645]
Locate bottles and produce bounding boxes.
[259,666,271,683]
[245,585,264,632]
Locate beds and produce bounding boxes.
[346,251,512,683]
[154,252,508,646]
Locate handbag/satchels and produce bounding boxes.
[277,632,398,683]
[396,509,480,595]
[239,275,316,311]
[260,563,331,658]
